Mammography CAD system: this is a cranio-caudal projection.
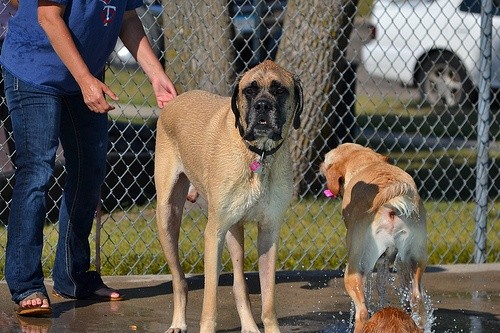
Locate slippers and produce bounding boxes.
[89,284,126,301]
[15,291,52,314]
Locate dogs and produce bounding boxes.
[154,58,304,333]
[318,143,430,332]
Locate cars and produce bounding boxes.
[363,0,499,109]
[112,0,288,70]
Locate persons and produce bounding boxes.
[1,0,179,315]
